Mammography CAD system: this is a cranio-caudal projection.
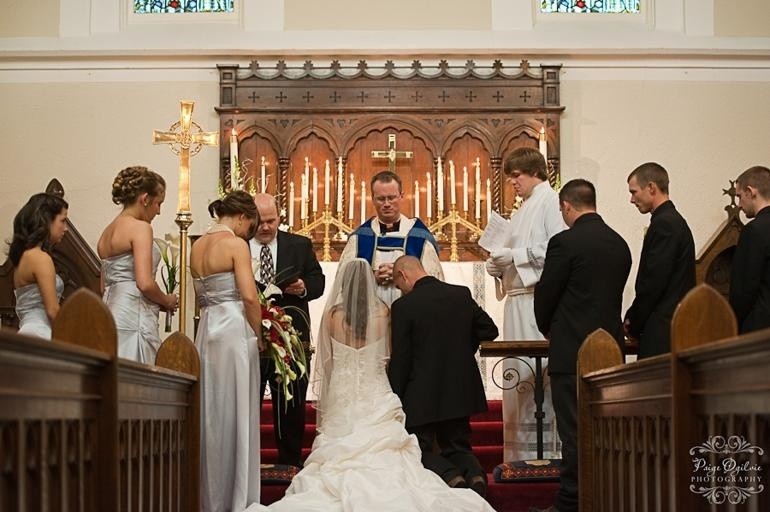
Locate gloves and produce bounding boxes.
[486,249,513,278]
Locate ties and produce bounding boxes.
[260,245,274,284]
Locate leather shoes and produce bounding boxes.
[448,476,487,498]
[528,505,559,512]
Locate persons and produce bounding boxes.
[385,254,499,499]
[249,192,326,469]
[189,191,266,512]
[243,258,496,511]
[96,166,179,367]
[529,178,632,512]
[5,193,68,342]
[487,146,570,463]
[337,171,446,310]
[727,166,770,337]
[622,162,697,361]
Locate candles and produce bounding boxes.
[213,136,548,227]
